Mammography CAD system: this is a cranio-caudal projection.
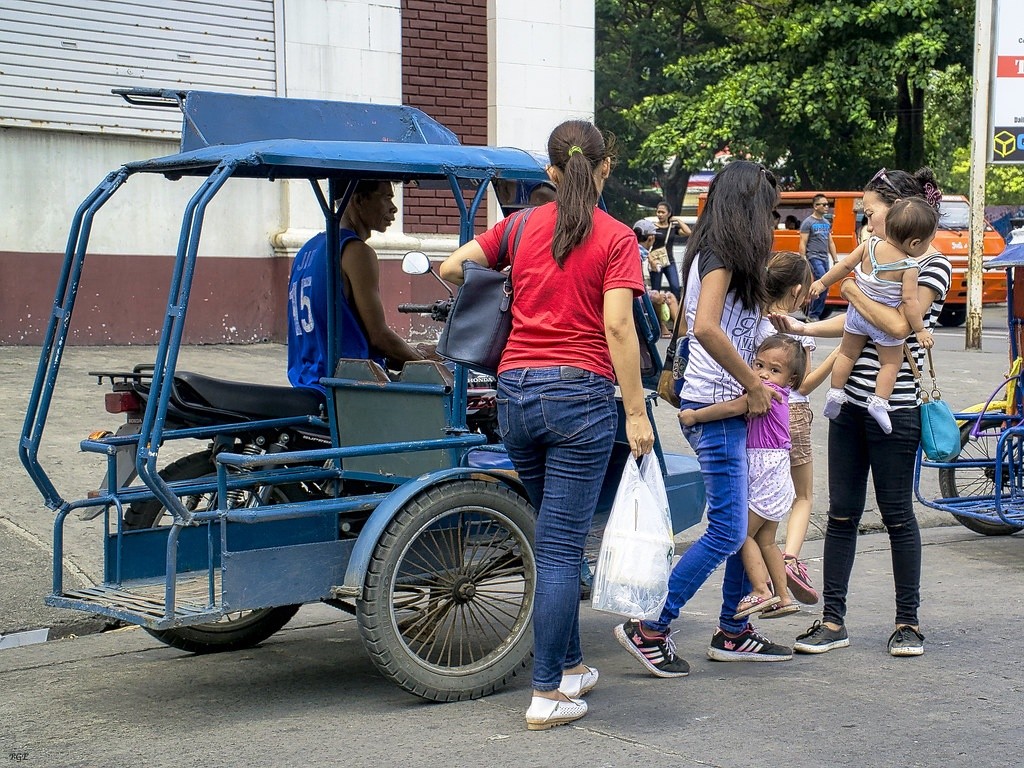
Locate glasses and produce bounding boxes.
[871,167,903,199]
[817,203,830,207]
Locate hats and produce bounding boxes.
[632,220,663,236]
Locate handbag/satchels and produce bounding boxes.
[647,246,672,269]
[657,335,690,409]
[591,447,675,621]
[434,208,535,375]
[920,399,961,460]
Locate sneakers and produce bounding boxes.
[614,618,690,677]
[707,622,792,661]
[783,554,819,605]
[793,620,849,653]
[887,625,925,655]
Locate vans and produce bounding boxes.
[693,192,1009,327]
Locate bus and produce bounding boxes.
[637,144,756,229]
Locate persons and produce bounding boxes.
[808,197,943,435]
[615,161,793,678]
[799,194,840,324]
[677,333,807,622]
[439,121,654,731]
[649,201,692,305]
[753,251,842,605]
[766,166,952,656]
[857,215,871,244]
[287,180,495,430]
[631,220,679,339]
[771,210,800,232]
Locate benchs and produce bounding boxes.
[328,358,515,484]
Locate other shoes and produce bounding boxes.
[558,664,599,698]
[526,692,588,730]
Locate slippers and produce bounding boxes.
[733,595,781,620]
[758,605,801,619]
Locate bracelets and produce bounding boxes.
[832,260,839,264]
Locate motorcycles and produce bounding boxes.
[17,83,708,704]
[915,243,1024,538]
